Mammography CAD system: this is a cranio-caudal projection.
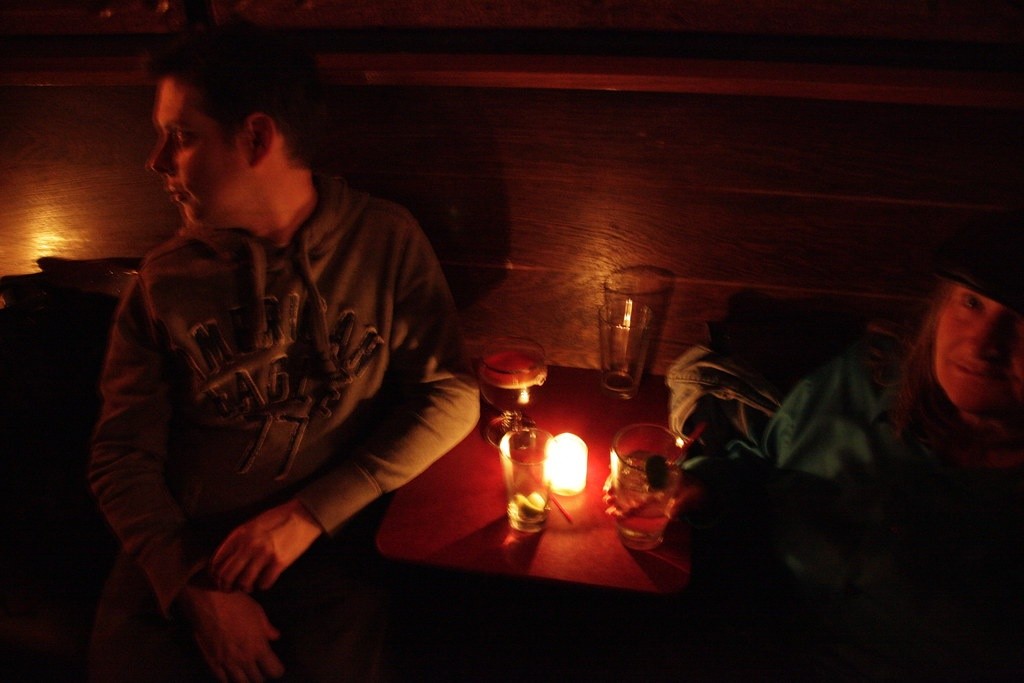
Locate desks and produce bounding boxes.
[376,366,709,601]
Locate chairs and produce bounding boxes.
[690,307,931,683]
[0,257,144,658]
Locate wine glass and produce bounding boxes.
[479,336,551,448]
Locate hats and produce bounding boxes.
[934,219,1024,319]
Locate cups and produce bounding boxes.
[500,428,556,532]
[610,423,686,551]
[598,300,652,396]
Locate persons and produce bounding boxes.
[685,214,1024,683]
[89,20,485,683]
